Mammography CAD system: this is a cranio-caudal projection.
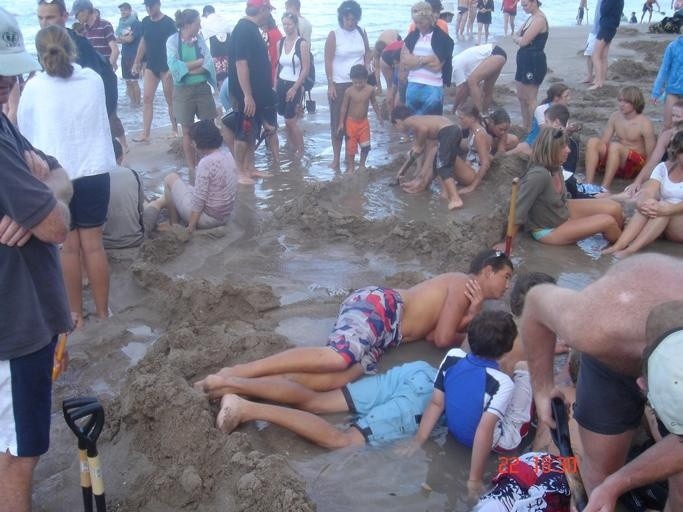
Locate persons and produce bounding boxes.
[157,0,312,243]
[0,0,159,512]
[195,248,682,512]
[325,0,683,257]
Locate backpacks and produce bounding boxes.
[279,36,316,91]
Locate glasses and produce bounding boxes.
[283,12,293,18]
[37,0,66,11]
[482,251,504,265]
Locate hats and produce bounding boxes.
[410,1,433,16]
[0,7,43,77]
[639,296,683,438]
[118,2,131,9]
[70,0,93,14]
[440,2,455,15]
[142,0,160,5]
[425,0,443,11]
[247,0,277,9]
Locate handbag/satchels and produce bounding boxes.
[221,110,259,142]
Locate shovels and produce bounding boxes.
[306,90,315,113]
